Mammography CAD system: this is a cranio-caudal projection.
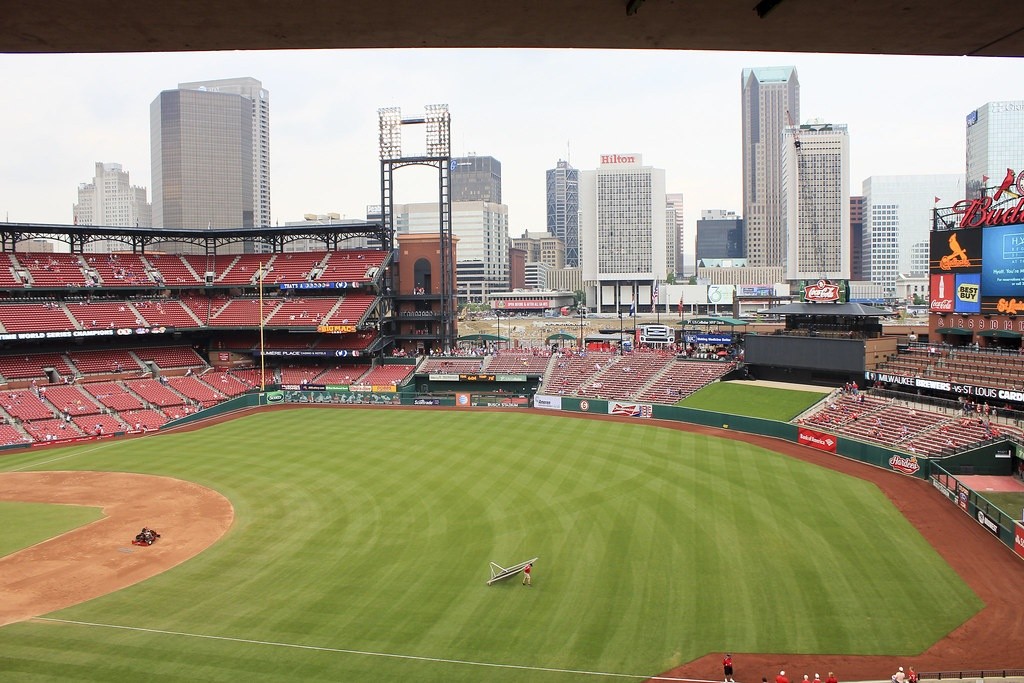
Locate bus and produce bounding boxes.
[560,306,571,316]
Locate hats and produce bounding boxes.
[899,667,903,671]
[804,675,808,679]
[828,672,834,677]
[815,673,820,678]
[780,671,785,675]
[727,654,730,656]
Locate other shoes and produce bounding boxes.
[730,678,735,682]
[523,583,525,585]
[725,679,728,683]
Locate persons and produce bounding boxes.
[825,671,837,683]
[0,251,1024,446]
[775,669,789,683]
[722,653,735,683]
[908,666,917,683]
[137,527,156,541]
[762,677,768,683]
[892,666,905,683]
[522,563,533,586]
[801,673,812,683]
[813,672,822,683]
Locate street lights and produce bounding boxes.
[495,311,500,352]
[618,308,623,355]
